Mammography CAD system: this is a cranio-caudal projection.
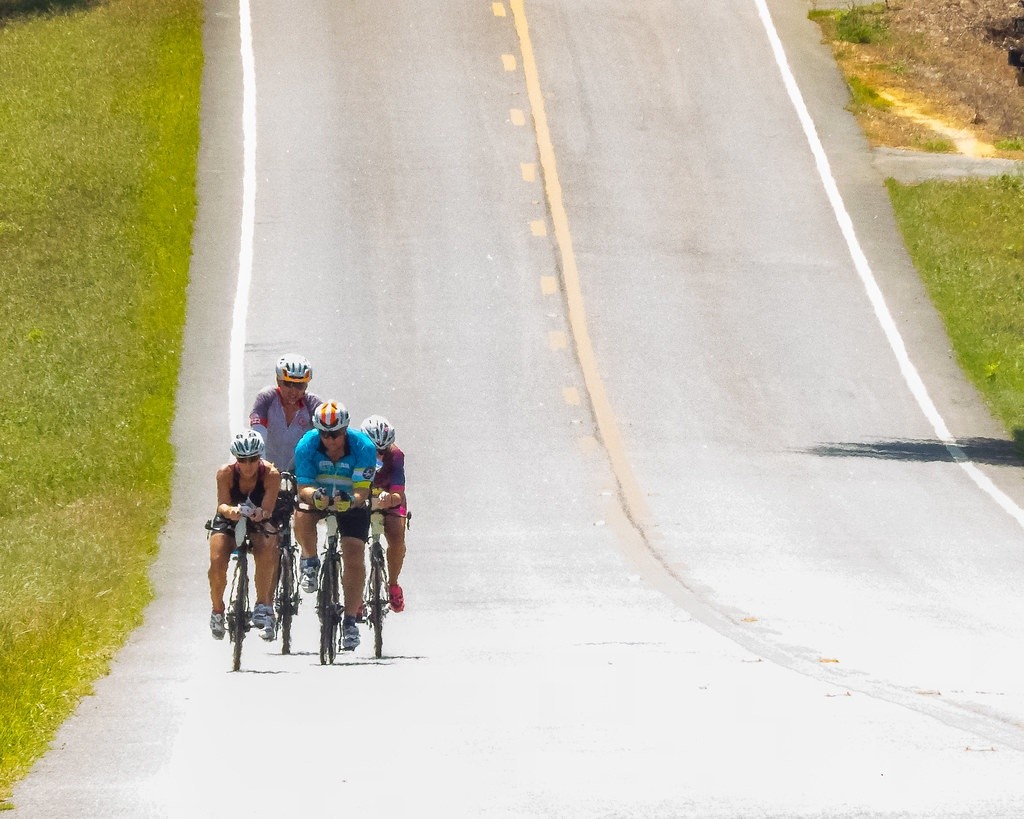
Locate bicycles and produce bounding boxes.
[204,507,286,670]
[367,504,412,660]
[276,471,298,655]
[293,489,372,666]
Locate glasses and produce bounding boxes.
[281,380,307,388]
[377,448,388,455]
[237,455,259,463]
[320,429,339,438]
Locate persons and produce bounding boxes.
[294,400,377,650]
[355,415,407,622]
[208,429,281,639]
[250,354,324,640]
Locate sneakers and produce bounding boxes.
[252,603,266,625]
[301,557,320,592]
[357,602,367,617]
[211,601,226,639]
[342,624,359,648]
[387,585,404,612]
[260,614,276,640]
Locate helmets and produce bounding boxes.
[361,415,395,449]
[276,353,312,382]
[312,400,350,430]
[230,430,265,458]
